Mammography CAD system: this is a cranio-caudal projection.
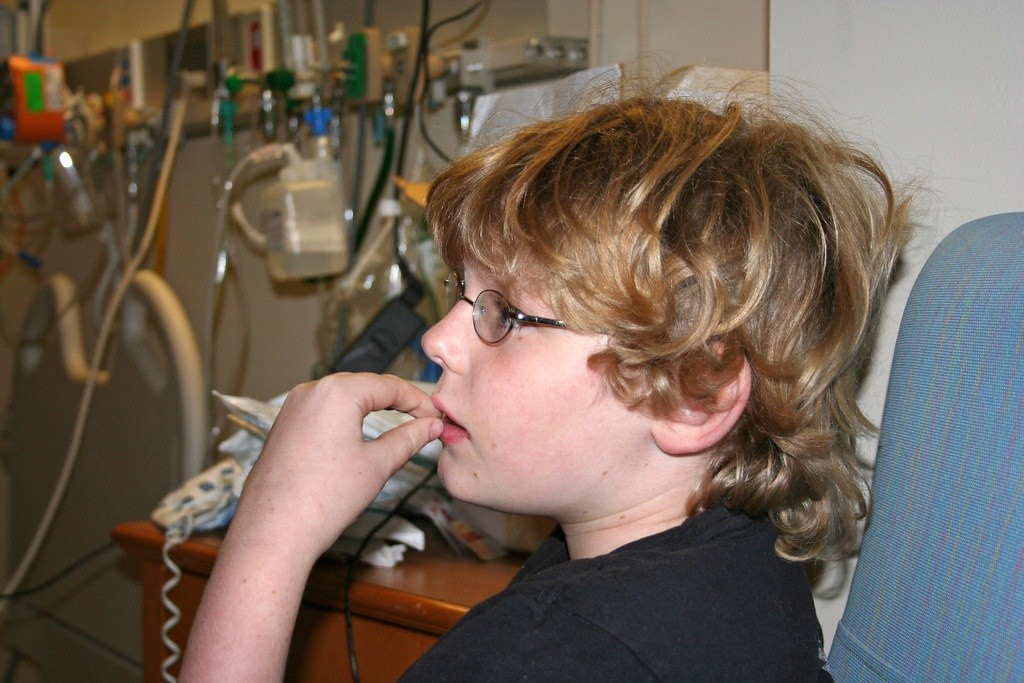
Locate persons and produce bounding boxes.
[176,75,918,683]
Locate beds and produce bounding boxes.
[802,211,1024,683]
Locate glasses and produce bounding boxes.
[443,271,616,344]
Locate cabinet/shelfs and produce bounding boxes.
[111,518,524,683]
